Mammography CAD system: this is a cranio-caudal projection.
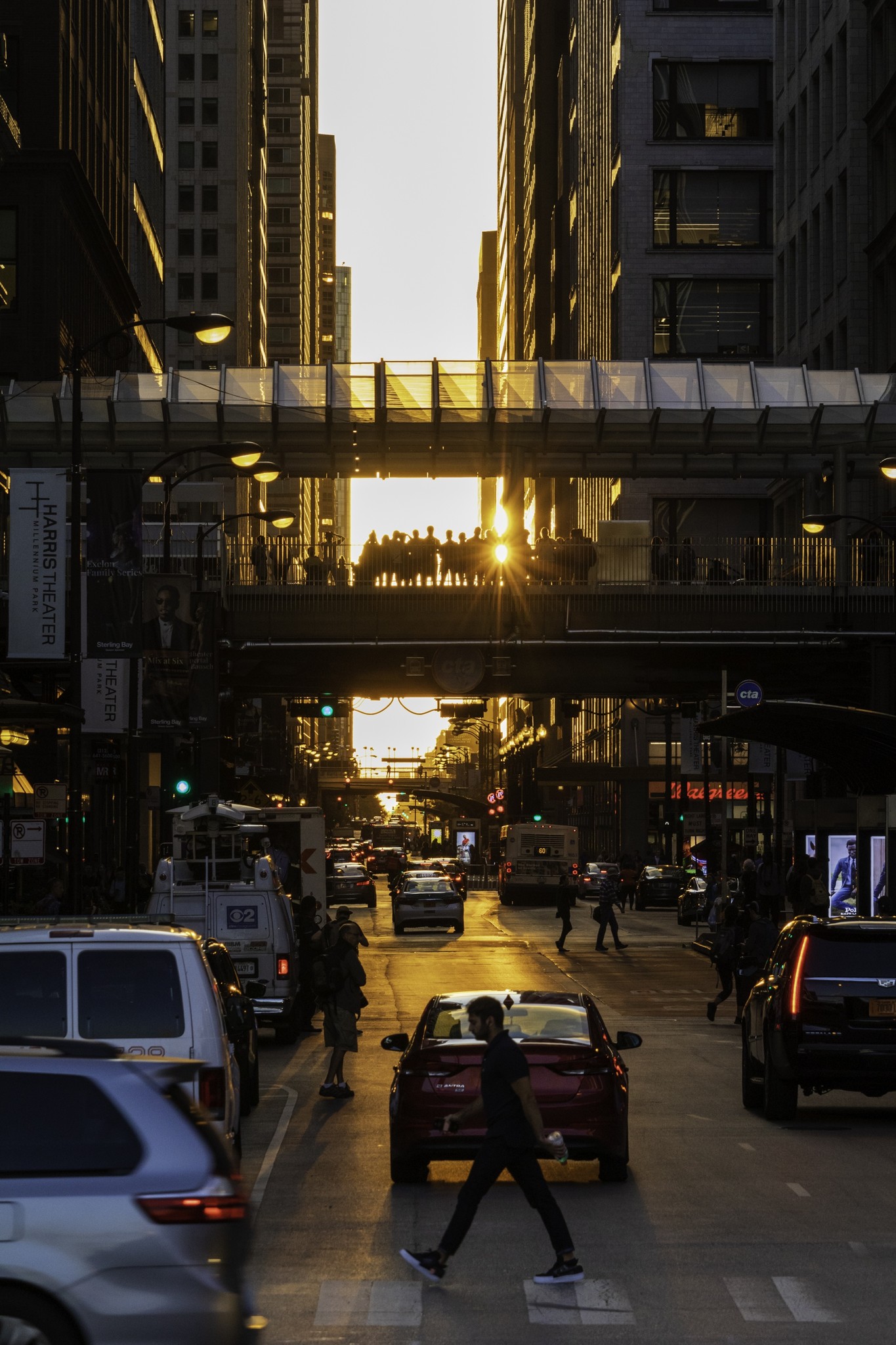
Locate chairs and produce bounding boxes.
[449,1023,476,1038]
[540,1019,574,1034]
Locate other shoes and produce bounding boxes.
[555,941,564,951]
[595,945,609,951]
[356,1029,363,1034]
[616,942,628,950]
[302,1025,322,1032]
[559,949,570,952]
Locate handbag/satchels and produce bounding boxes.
[556,912,560,918]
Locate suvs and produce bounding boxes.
[200,937,268,1116]
[740,912,895,1122]
[1,1033,260,1345]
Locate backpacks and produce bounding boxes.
[710,925,740,968]
[806,873,830,908]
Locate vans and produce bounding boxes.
[152,855,307,1045]
[1,916,248,1191]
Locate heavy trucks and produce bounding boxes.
[168,794,329,968]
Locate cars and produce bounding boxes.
[378,989,643,1181]
[635,865,688,910]
[325,813,469,908]
[578,862,622,901]
[677,877,738,927]
[393,879,466,934]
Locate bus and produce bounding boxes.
[497,824,580,907]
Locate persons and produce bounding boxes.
[831,840,857,915]
[145,585,195,648]
[556,849,639,952]
[705,851,832,1025]
[296,896,369,1098]
[31,858,153,918]
[469,844,484,880]
[388,833,456,892]
[387,763,424,778]
[251,526,701,585]
[462,834,472,863]
[396,995,586,1287]
[873,862,886,915]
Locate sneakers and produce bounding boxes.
[400,1248,449,1282]
[707,1002,717,1021]
[338,1080,354,1098]
[734,1016,742,1023]
[533,1257,584,1284]
[319,1082,340,1097]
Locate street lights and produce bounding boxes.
[63,307,300,914]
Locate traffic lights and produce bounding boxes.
[497,806,504,813]
[337,797,342,807]
[488,808,495,815]
[169,776,196,801]
[346,778,350,788]
[533,814,544,822]
[388,780,393,790]
[320,704,334,717]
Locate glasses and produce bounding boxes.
[335,914,339,919]
[347,933,360,936]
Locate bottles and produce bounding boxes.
[548,1131,569,1165]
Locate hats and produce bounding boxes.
[606,868,620,877]
[337,906,353,915]
